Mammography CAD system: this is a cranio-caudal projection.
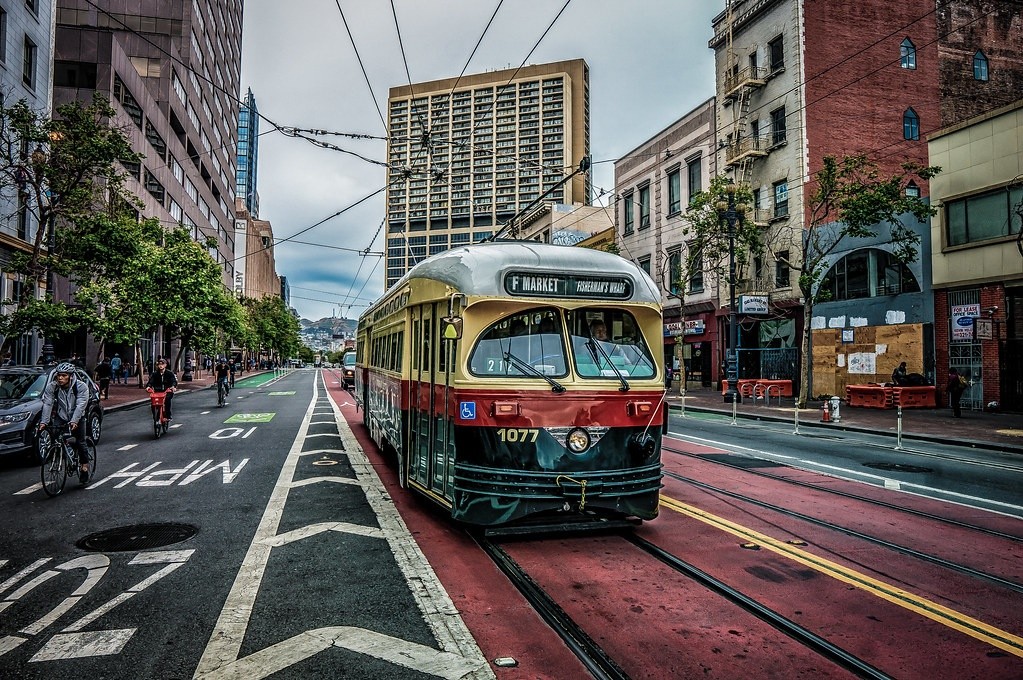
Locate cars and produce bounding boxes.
[0,364,104,466]
[341,351,356,390]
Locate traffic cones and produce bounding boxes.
[820,400,832,422]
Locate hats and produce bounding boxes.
[158,359,167,364]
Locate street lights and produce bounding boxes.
[31,130,66,366]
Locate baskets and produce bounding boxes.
[149,392,166,405]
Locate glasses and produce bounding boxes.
[222,363,226,364]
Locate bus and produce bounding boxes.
[357,244,668,538]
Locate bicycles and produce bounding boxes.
[218,382,229,406]
[41,424,97,496]
[145,388,170,436]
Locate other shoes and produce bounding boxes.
[80,470,89,483]
[163,418,170,422]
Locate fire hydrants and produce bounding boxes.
[828,396,842,423]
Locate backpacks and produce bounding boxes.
[957,374,968,390]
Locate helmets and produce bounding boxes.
[220,358,227,363]
[56,362,75,373]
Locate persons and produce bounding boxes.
[685,365,689,391]
[39,362,90,484]
[111,353,121,384]
[892,362,906,384]
[946,367,964,417]
[123,363,132,384]
[146,359,178,422]
[145,355,153,375]
[94,357,111,398]
[215,358,230,404]
[1,352,16,366]
[206,358,212,372]
[228,359,237,387]
[576,320,631,364]
[193,358,196,373]
[666,364,673,392]
[70,353,83,368]
